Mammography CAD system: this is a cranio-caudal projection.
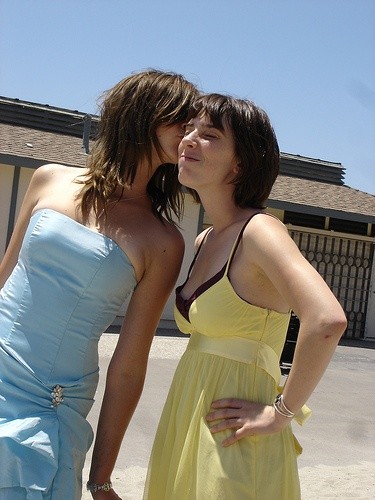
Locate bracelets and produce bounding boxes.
[86,481,112,492]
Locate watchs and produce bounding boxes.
[273,394,296,418]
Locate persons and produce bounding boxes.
[0,67,203,500]
[143,94,347,500]
